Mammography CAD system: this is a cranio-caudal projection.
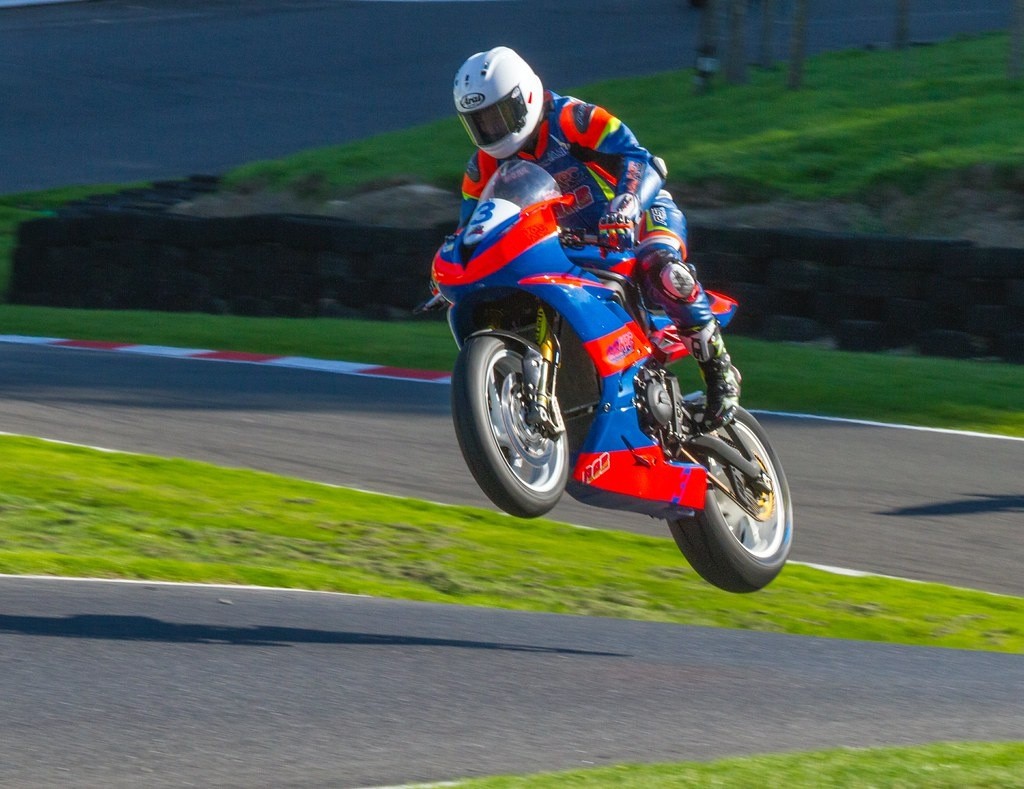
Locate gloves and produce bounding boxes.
[598,213,637,253]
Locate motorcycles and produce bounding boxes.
[412,155,793,594]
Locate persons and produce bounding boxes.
[427,46,741,432]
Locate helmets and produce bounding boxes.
[451,46,545,159]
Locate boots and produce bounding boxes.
[675,314,742,434]
